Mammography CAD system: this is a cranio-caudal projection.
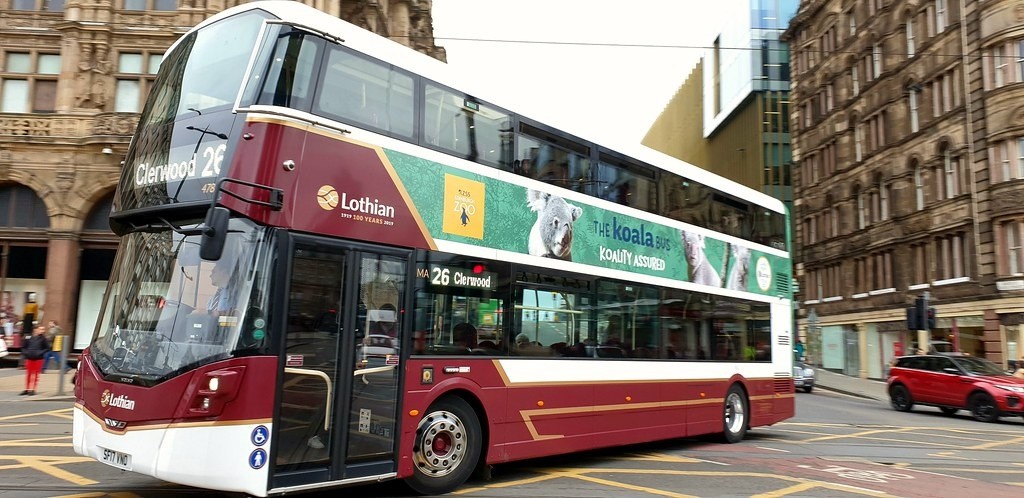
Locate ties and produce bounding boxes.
[211,288,220,313]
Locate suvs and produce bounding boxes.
[885,351,1024,423]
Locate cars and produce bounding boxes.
[793,360,815,393]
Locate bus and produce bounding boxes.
[74,3,794,497]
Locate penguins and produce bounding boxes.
[459,207,470,227]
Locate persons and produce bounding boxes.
[0,292,17,330]
[453,318,657,359]
[511,153,576,187]
[364,302,401,349]
[20,326,49,397]
[20,292,39,339]
[189,258,242,318]
[1012,361,1024,379]
[34,324,51,361]
[42,321,71,375]
[915,348,923,356]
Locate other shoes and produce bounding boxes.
[65,367,72,373]
[20,390,37,396]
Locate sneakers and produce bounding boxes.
[307,436,326,449]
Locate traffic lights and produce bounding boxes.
[906,294,936,330]
